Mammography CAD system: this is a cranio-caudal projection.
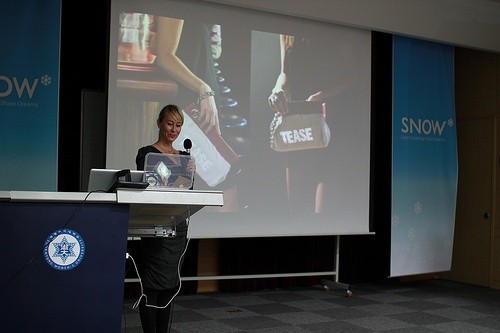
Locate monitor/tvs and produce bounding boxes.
[87,169,144,193]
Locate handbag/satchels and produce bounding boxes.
[265,98,331,153]
[168,102,239,187]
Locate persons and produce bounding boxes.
[269,33,347,215]
[132,104,197,332]
[151,15,242,215]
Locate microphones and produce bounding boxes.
[183,139,192,155]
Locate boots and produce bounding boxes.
[158,287,179,333]
[139,285,159,333]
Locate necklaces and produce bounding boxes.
[156,141,176,155]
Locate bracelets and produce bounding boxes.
[196,90,215,103]
